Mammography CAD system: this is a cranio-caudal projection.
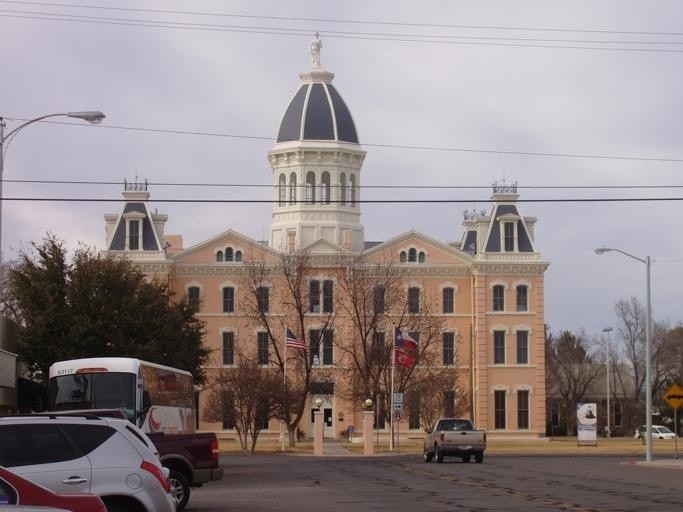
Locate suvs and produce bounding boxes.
[0,414,178,512]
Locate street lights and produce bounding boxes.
[602,324,616,438]
[0,108,111,224]
[591,245,656,462]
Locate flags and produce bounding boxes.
[393,324,418,350]
[284,328,308,352]
[393,348,417,370]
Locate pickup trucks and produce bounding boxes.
[423,416,488,463]
[13,409,225,512]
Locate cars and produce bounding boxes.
[633,420,678,439]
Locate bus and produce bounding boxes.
[29,357,198,434]
